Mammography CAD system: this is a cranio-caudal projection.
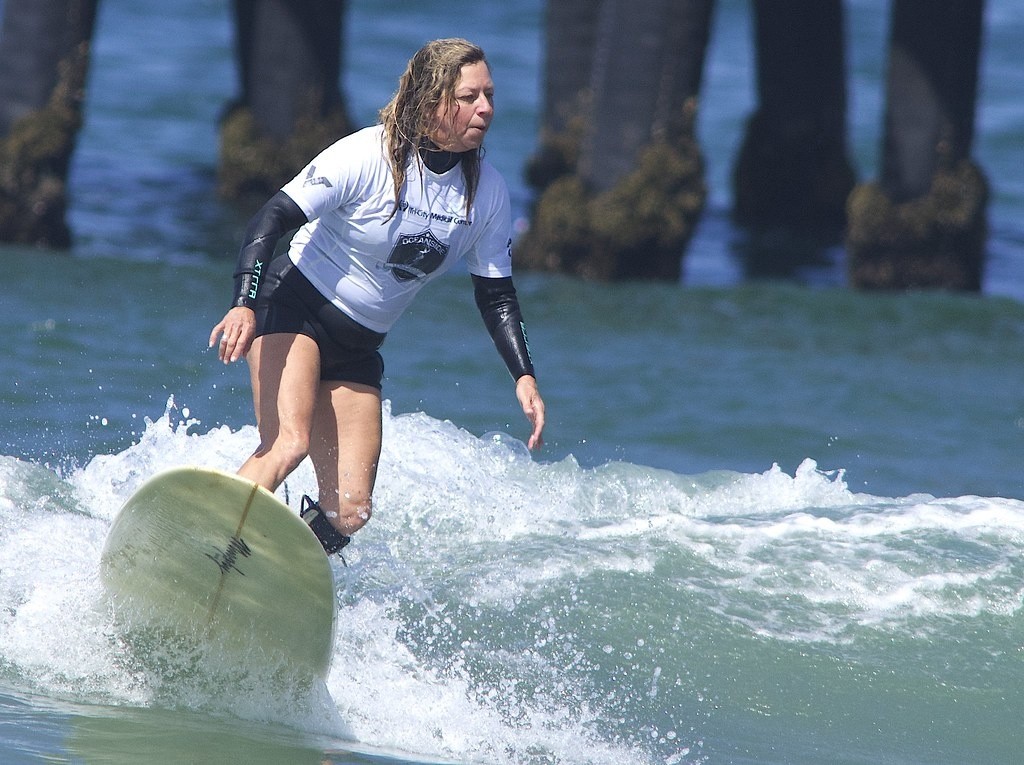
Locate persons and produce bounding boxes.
[209,37,545,558]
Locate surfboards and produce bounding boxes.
[98,465,337,692]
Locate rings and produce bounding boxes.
[221,335,229,340]
